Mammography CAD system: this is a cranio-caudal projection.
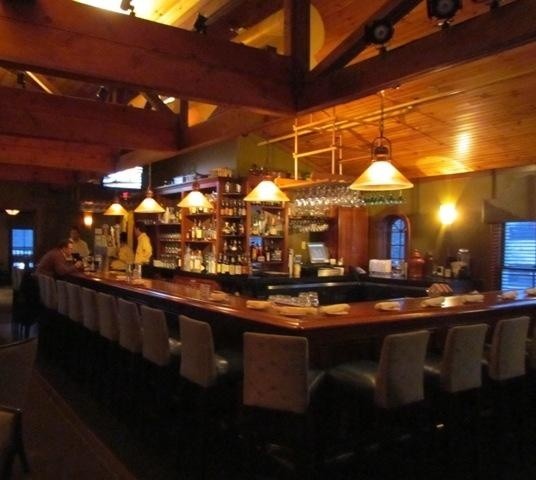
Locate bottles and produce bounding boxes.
[436,260,452,279]
[293,262,300,278]
[136,188,283,278]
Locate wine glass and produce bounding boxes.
[126,263,134,284]
[287,184,404,233]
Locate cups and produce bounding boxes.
[299,292,320,307]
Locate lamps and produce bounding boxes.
[104,191,128,216]
[244,139,291,203]
[132,162,166,213]
[346,89,414,190]
[177,170,213,209]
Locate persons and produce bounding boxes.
[114,232,133,270]
[133,222,154,279]
[37,239,82,278]
[68,229,89,262]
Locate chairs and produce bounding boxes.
[327,327,431,477]
[483,315,530,479]
[525,320,536,372]
[426,324,491,479]
[0,403,30,476]
[242,331,324,479]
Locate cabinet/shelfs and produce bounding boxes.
[242,175,289,275]
[283,175,368,273]
[139,175,249,274]
[360,280,429,302]
[265,281,361,305]
